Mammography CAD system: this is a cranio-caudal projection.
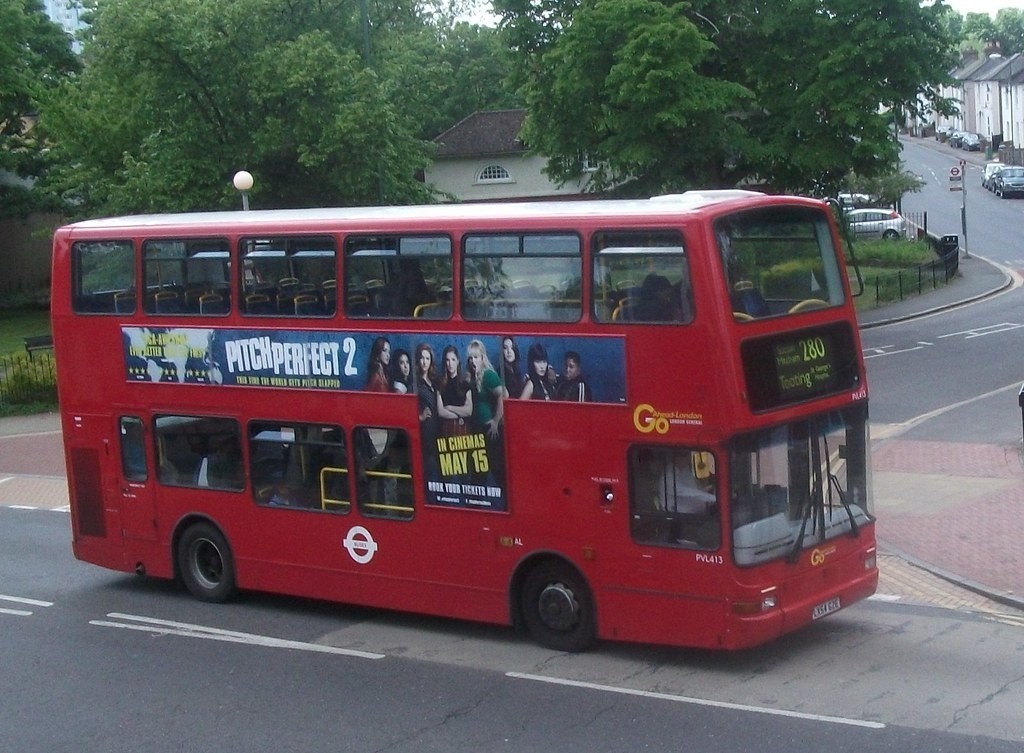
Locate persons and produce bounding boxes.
[372,441,408,513]
[660,449,716,512]
[269,475,305,507]
[364,336,593,506]
[635,273,684,321]
[191,434,245,490]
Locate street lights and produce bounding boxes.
[989,53,1013,146]
[234,170,254,252]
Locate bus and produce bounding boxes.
[50,189,878,652]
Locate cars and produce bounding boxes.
[995,167,1024,199]
[935,126,957,143]
[962,134,987,151]
[948,131,970,148]
[981,164,1006,189]
[838,193,876,214]
[989,171,998,193]
[847,209,906,241]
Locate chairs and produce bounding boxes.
[255,486,274,504]
[115,279,775,319]
